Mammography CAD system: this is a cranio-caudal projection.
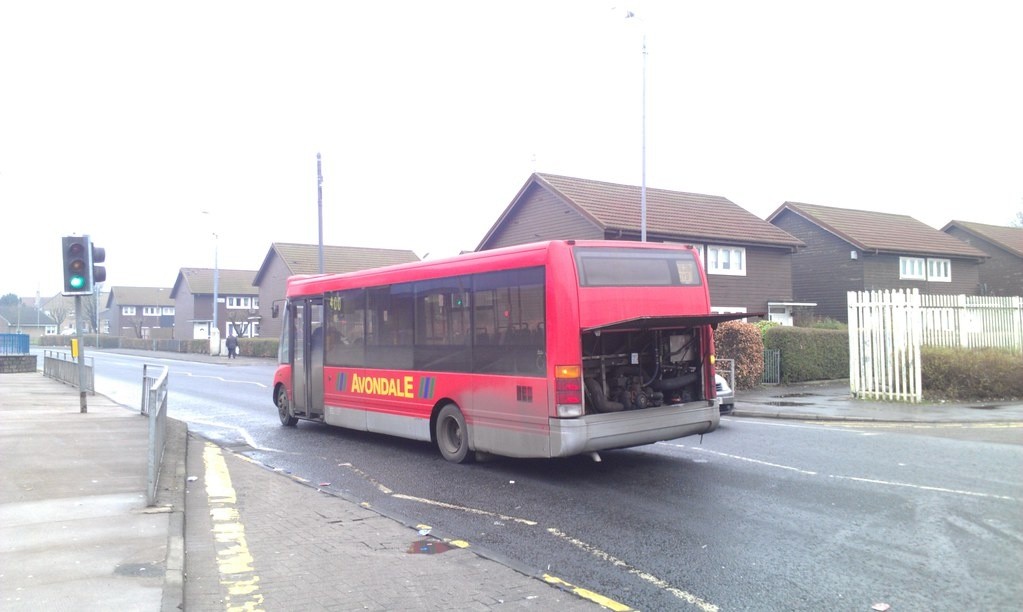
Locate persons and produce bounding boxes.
[226,333,239,358]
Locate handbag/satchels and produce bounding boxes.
[235,346,240,355]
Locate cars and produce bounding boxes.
[715,374,734,416]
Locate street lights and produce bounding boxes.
[612,5,647,243]
[202,211,221,355]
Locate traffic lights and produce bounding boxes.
[60,234,106,296]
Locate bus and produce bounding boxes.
[272,240,768,464]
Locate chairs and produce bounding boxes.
[337,320,545,348]
